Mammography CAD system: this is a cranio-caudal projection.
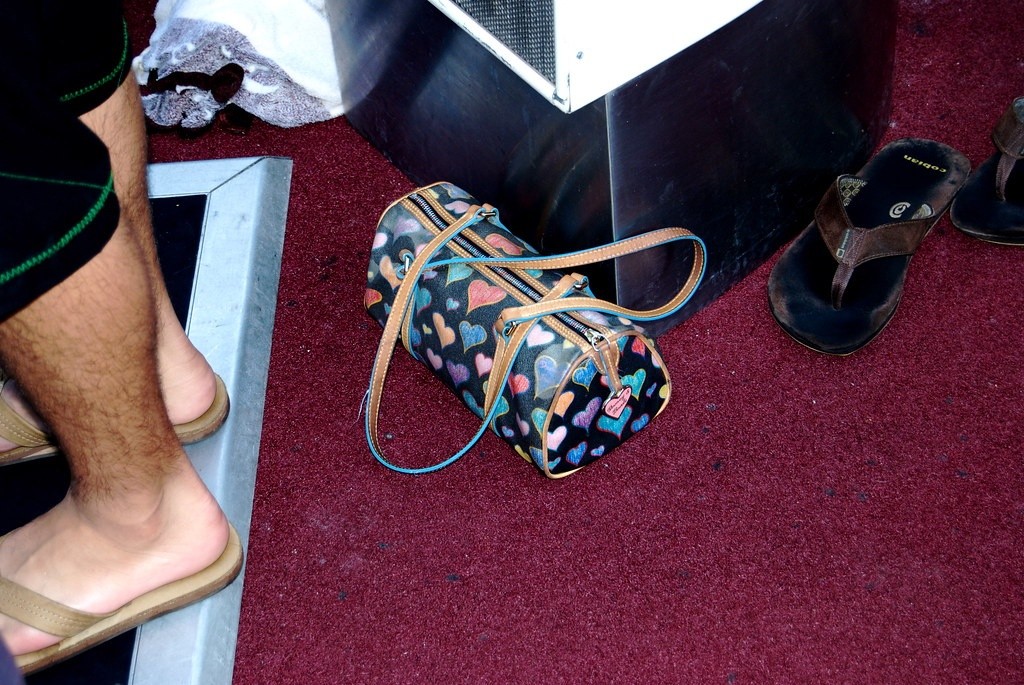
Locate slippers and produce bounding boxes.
[0,372,230,467]
[949,98,1024,245]
[0,522,244,676]
[769,139,973,355]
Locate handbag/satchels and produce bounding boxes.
[356,182,706,478]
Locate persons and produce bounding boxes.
[0,0,244,674]
